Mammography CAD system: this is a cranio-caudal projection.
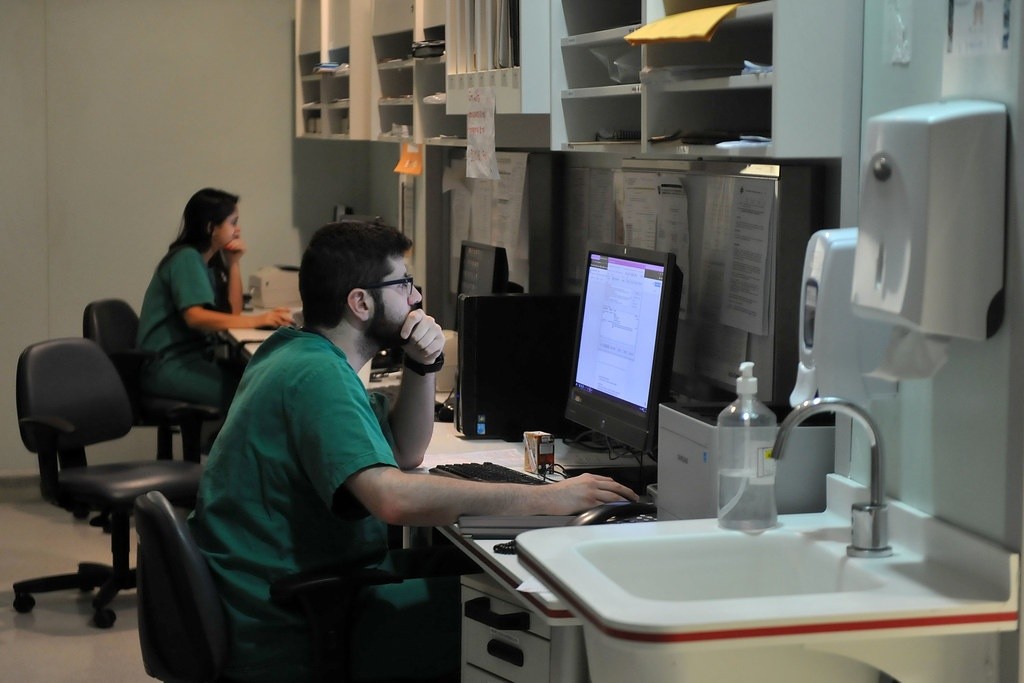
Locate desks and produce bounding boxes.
[226,305,653,683]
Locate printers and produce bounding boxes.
[248,264,305,312]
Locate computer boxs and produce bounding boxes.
[454,292,581,439]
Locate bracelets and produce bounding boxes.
[404,352,444,376]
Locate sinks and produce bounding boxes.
[572,531,885,602]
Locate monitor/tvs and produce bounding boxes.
[334,205,353,224]
[564,238,683,494]
[454,240,524,328]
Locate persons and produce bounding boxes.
[185,223,640,683]
[136,188,297,405]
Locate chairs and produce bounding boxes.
[12,337,207,629]
[133,490,442,683]
[84,300,223,465]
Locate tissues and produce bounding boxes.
[850,97,1008,384]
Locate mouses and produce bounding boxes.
[255,325,279,331]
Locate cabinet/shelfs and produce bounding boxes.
[548,0,865,160]
[295,0,549,150]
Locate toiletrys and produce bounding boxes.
[714,361,778,536]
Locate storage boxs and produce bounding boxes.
[249,264,305,308]
[435,330,460,393]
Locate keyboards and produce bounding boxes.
[427,462,554,485]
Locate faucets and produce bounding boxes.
[770,397,893,557]
